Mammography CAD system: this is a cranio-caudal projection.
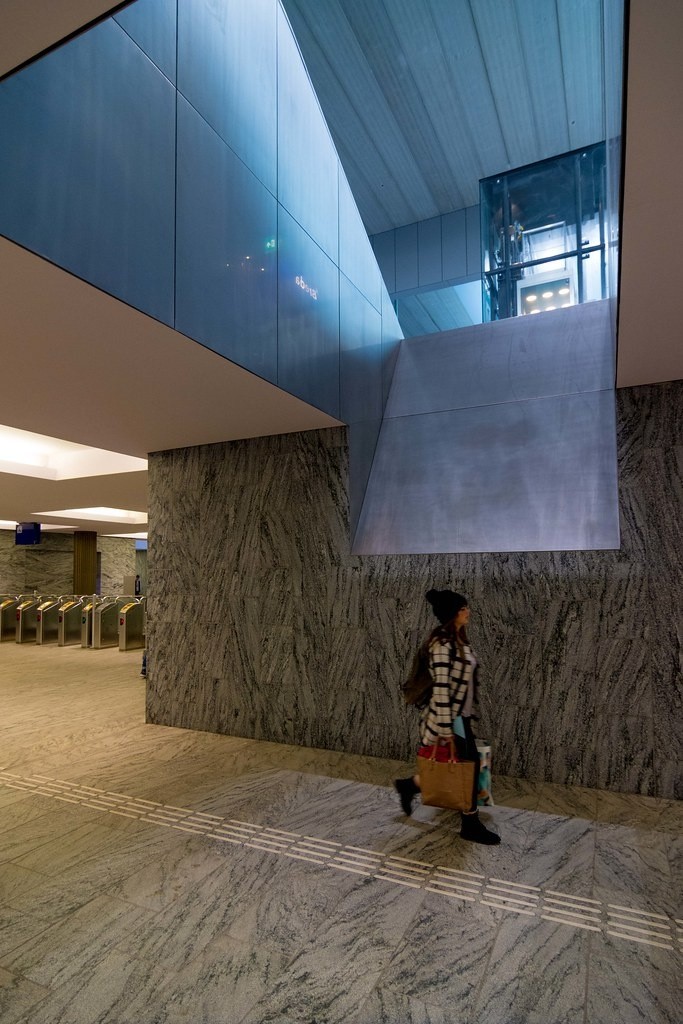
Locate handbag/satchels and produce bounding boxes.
[474,737,495,807]
[416,735,473,812]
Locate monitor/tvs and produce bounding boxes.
[16,524,41,545]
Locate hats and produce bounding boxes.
[425,588,466,624]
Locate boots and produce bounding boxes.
[393,774,420,817]
[460,808,501,845]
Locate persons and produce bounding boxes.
[394,588,502,845]
[140,654,146,677]
[135,575,141,600]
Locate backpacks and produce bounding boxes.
[404,633,457,709]
